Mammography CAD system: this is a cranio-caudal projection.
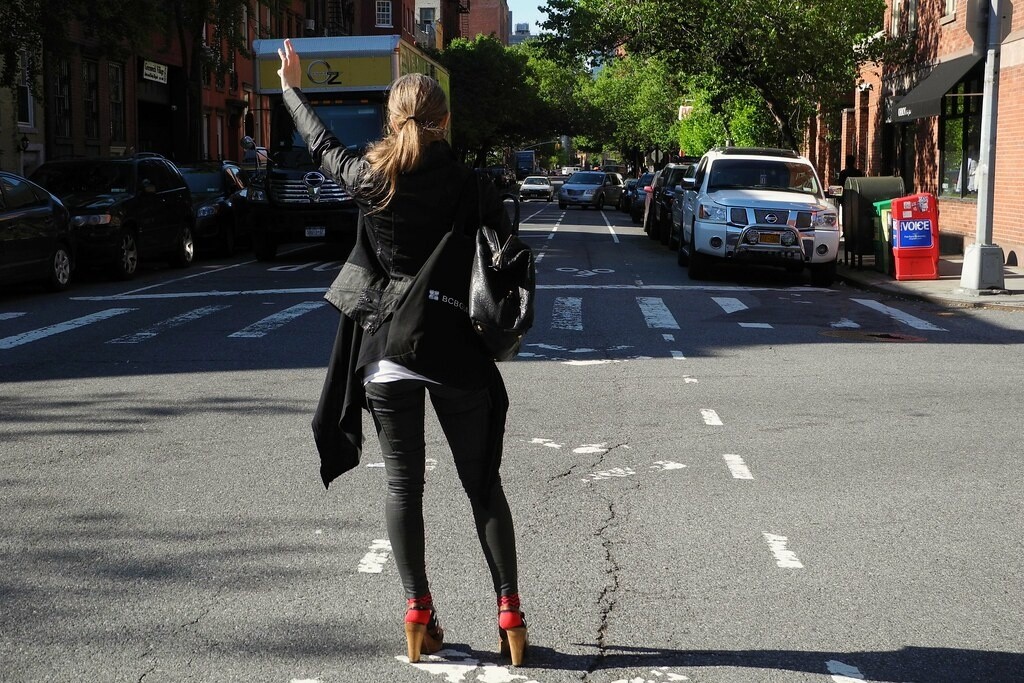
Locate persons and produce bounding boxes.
[956,137,979,194]
[278,38,527,666]
[838,155,863,187]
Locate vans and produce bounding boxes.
[561,167,581,176]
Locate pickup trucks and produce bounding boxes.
[518,176,554,202]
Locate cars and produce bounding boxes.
[601,165,626,174]
[474,165,516,188]
[558,172,624,210]
[0,170,76,291]
[619,162,700,251]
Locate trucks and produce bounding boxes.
[238,34,451,263]
[513,150,537,179]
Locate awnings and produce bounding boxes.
[891,54,986,123]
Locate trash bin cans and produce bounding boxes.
[843,177,906,270]
[890,193,941,281]
[872,198,892,275]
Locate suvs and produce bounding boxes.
[174,160,253,256]
[26,152,196,281]
[681,147,839,287]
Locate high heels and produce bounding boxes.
[404,602,443,663]
[497,604,528,667]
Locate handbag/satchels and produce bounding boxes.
[468,224,536,361]
